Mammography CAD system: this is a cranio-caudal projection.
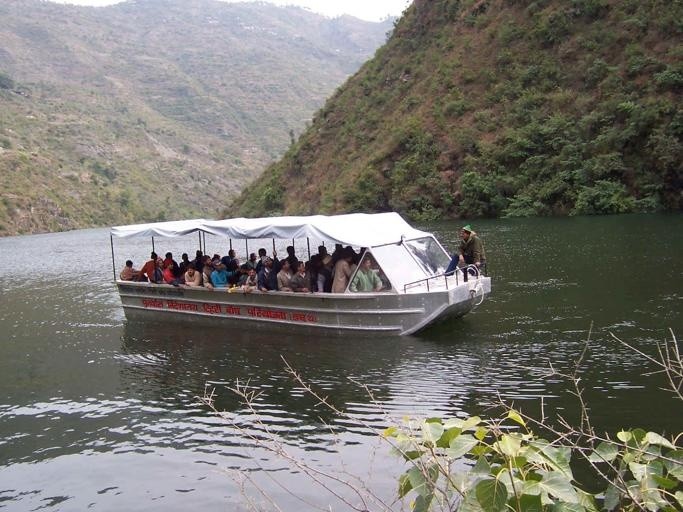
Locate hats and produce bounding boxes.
[463,225,472,232]
[262,257,269,263]
[212,259,221,266]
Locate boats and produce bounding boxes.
[106,210,493,335]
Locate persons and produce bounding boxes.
[119,242,391,295]
[443,225,486,277]
